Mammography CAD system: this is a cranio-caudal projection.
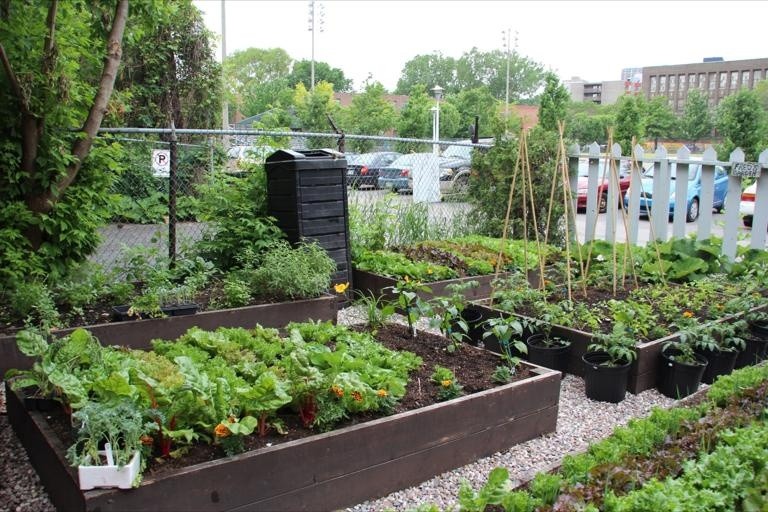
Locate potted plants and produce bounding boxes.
[437,278,767,405]
[112,239,215,322]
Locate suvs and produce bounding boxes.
[439,141,501,201]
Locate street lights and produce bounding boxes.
[428,106,440,152]
[305,1,325,90]
[431,84,445,153]
[500,26,520,139]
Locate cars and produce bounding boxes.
[221,145,275,177]
[740,179,757,227]
[345,151,403,190]
[576,158,631,213]
[623,161,728,222]
[376,153,430,195]
